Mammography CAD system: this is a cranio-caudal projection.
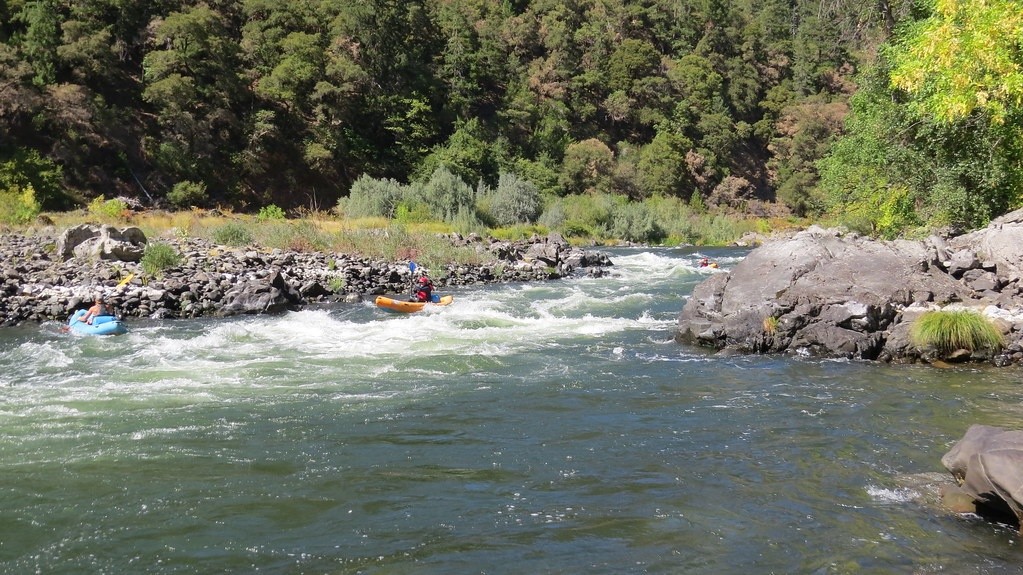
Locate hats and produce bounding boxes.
[94,294,104,303]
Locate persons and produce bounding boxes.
[700,258,708,268]
[77,294,113,325]
[408,278,433,302]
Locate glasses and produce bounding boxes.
[419,282,425,283]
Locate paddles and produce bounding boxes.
[408,261,415,296]
[64,274,133,330]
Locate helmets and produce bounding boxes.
[419,278,428,283]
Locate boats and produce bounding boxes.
[375,295,454,314]
[707,263,723,269]
[68,309,129,336]
